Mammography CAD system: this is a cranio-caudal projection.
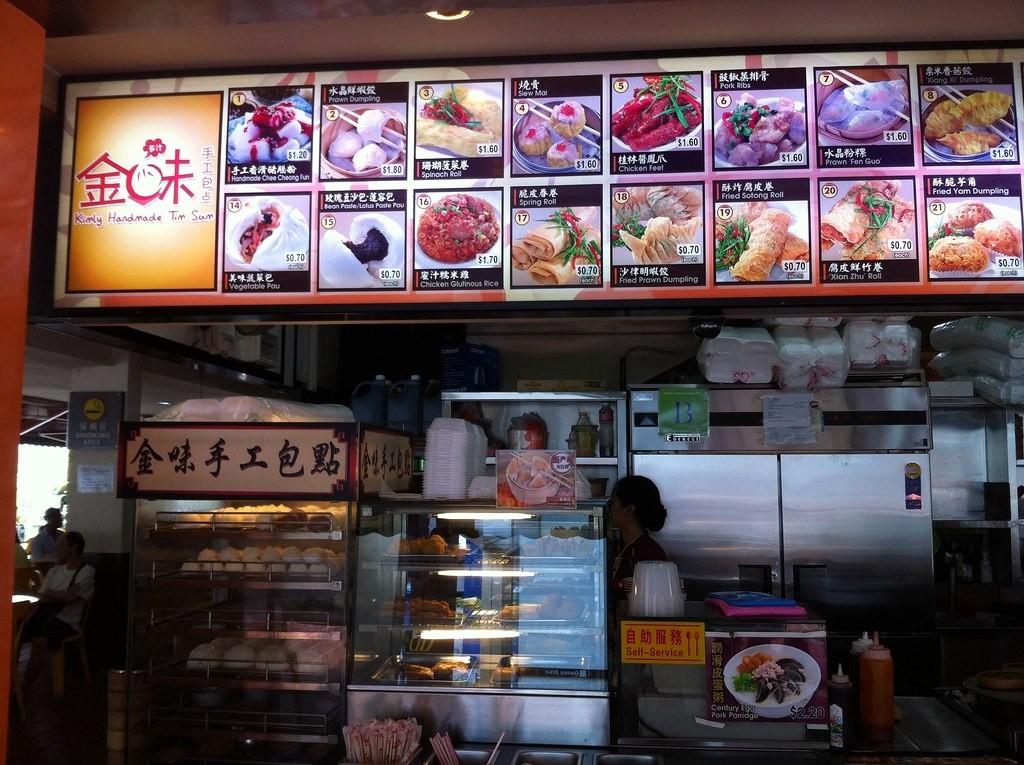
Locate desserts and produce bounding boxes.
[368,536,595,681]
[926,201,1022,279]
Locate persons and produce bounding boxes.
[10,529,97,705]
[13,506,66,591]
[605,473,669,703]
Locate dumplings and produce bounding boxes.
[924,90,1013,157]
[818,78,904,131]
[614,184,703,265]
[329,109,388,173]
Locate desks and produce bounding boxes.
[9,592,47,719]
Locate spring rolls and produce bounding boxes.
[415,90,499,156]
[822,180,913,260]
[511,207,602,285]
[714,201,809,282]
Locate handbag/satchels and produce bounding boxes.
[27,600,67,627]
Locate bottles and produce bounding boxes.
[350,375,442,436]
[847,632,876,680]
[828,662,857,749]
[599,403,615,457]
[861,632,894,742]
[577,411,591,425]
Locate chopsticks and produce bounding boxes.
[827,70,910,122]
[323,104,406,155]
[510,452,573,489]
[515,98,601,149]
[934,85,1016,146]
[341,722,422,765]
[428,731,506,765]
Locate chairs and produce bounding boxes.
[12,568,98,719]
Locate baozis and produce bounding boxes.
[178,546,343,674]
[226,198,309,271]
[316,213,406,288]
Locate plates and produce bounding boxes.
[623,577,687,605]
[415,198,502,268]
[713,108,806,167]
[613,96,703,151]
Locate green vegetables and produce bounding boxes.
[732,672,757,691]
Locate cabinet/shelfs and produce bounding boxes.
[441,391,628,499]
[931,400,1024,587]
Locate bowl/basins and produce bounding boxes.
[625,561,685,617]
[512,102,601,173]
[723,644,821,718]
[588,477,609,497]
